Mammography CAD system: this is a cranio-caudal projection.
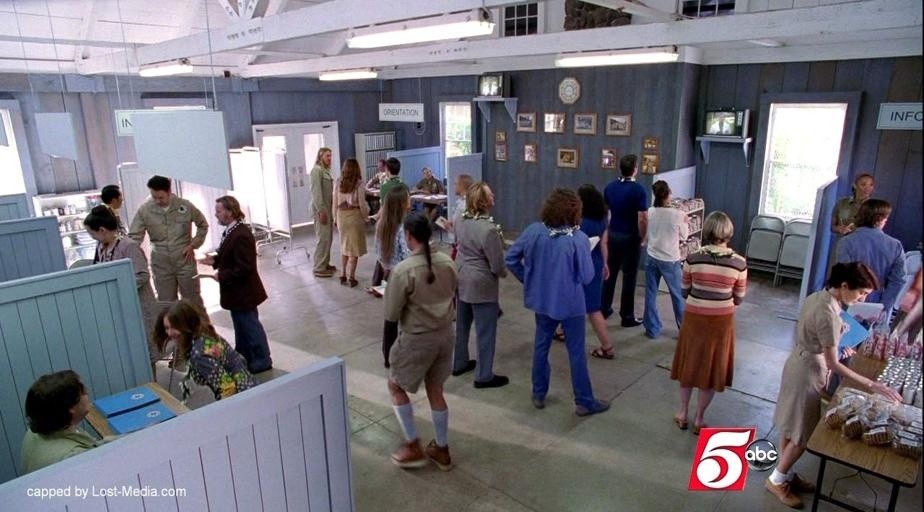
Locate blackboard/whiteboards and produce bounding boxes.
[132,111,234,191]
[34,112,75,159]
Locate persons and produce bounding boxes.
[153,299,273,399]
[126,175,210,328]
[893,267,923,341]
[762,263,902,508]
[193,195,272,373]
[827,173,875,281]
[98,185,126,238]
[19,370,125,475]
[84,205,160,383]
[818,199,905,402]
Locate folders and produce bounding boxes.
[93,385,178,435]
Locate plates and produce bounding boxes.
[59,232,97,249]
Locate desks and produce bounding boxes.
[801,347,923,512]
[83,381,193,441]
[364,188,448,245]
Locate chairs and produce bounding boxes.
[154,298,185,393]
[744,214,787,289]
[771,219,812,286]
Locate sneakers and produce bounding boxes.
[530,394,547,410]
[673,414,691,430]
[390,438,427,472]
[574,398,611,417]
[313,264,336,278]
[425,439,452,473]
[690,421,709,436]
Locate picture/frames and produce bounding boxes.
[542,110,568,135]
[572,111,598,136]
[640,135,660,175]
[555,145,579,169]
[516,110,538,133]
[604,111,633,137]
[523,140,540,163]
[600,145,619,171]
[494,129,508,162]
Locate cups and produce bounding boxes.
[876,355,923,409]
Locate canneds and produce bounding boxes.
[876,355,923,408]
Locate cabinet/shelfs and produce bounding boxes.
[353,131,397,189]
[30,187,109,271]
[680,195,706,268]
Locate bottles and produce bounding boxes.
[38,195,99,233]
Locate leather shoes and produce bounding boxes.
[764,473,817,507]
[451,358,477,376]
[621,316,644,328]
[474,374,511,390]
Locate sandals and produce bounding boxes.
[550,328,567,342]
[590,345,616,359]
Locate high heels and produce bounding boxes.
[339,274,358,287]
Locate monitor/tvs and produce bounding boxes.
[474,72,510,98]
[703,109,750,138]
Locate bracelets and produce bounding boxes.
[867,380,873,393]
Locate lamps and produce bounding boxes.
[554,44,680,67]
[138,56,194,77]
[317,68,379,82]
[343,7,497,52]
